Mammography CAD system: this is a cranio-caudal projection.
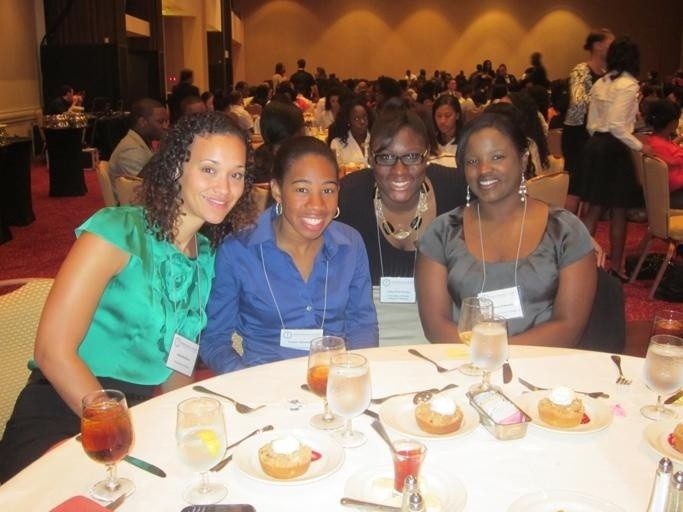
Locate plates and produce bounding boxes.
[644,419,683,463]
[517,389,613,433]
[240,429,338,482]
[380,395,480,437]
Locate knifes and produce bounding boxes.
[300,383,379,418]
[516,377,609,400]
[227,422,274,450]
[502,361,512,384]
[372,420,397,452]
[76,435,166,478]
[663,389,683,406]
[368,389,442,404]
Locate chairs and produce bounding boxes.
[95,161,118,207]
[0,278,56,444]
[547,155,565,173]
[525,171,569,207]
[113,175,144,206]
[626,154,683,301]
[248,183,268,211]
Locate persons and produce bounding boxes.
[108,97,168,206]
[561,28,616,223]
[169,68,201,122]
[316,88,340,129]
[585,34,661,284]
[308,81,320,100]
[333,107,479,284]
[639,98,683,194]
[0,110,255,484]
[197,136,380,376]
[272,62,287,96]
[201,92,215,110]
[426,94,468,168]
[290,59,314,93]
[414,112,599,348]
[329,51,550,112]
[314,66,327,96]
[327,99,373,180]
[42,84,83,169]
[250,85,269,104]
[236,80,248,97]
[251,100,306,155]
[182,96,206,115]
[227,91,254,129]
[640,66,683,108]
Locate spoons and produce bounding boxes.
[413,384,458,404]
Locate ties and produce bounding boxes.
[451,91,454,96]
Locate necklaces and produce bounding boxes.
[373,179,432,241]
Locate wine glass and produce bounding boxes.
[308,336,346,428]
[457,297,492,376]
[466,314,508,397]
[82,389,135,501]
[650,311,683,339]
[326,353,370,447]
[177,398,225,506]
[641,335,683,422]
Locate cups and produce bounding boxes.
[393,441,426,491]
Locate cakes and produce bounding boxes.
[257,437,312,479]
[412,395,464,434]
[668,423,682,451]
[538,385,584,429]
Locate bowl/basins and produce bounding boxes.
[469,389,531,440]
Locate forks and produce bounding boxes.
[611,355,631,386]
[193,385,265,414]
[408,349,457,374]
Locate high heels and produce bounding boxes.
[607,267,631,285]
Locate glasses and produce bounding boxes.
[371,148,428,167]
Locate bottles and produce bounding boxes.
[401,475,426,512]
[645,456,675,512]
[669,468,680,511]
